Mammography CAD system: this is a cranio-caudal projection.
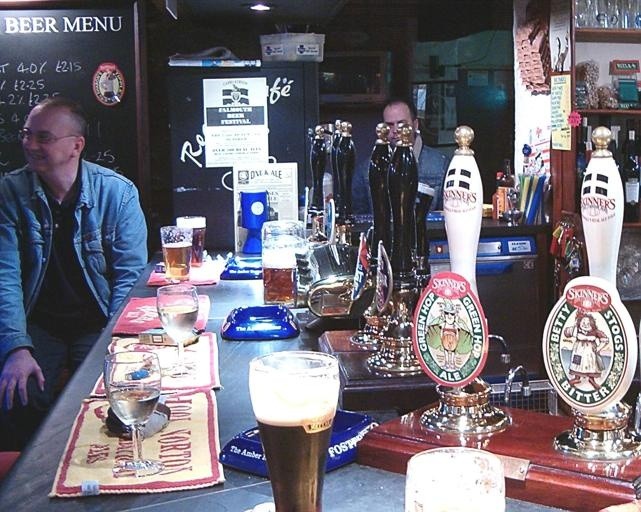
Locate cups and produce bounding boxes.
[406,446,507,511]
[159,216,207,280]
[572,1,641,28]
[262,220,308,306]
[249,350,341,511]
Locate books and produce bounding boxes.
[168,58,262,69]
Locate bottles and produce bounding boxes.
[577,109,641,224]
[492,158,514,221]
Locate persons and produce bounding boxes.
[0,93,148,454]
[345,93,451,213]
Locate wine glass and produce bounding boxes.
[105,285,200,476]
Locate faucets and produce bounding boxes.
[505,365,532,407]
[489,333,510,364]
[633,391,641,436]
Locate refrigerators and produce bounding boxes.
[166,53,322,251]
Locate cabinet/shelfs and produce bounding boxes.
[547,2,641,419]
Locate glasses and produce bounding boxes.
[17,127,80,144]
